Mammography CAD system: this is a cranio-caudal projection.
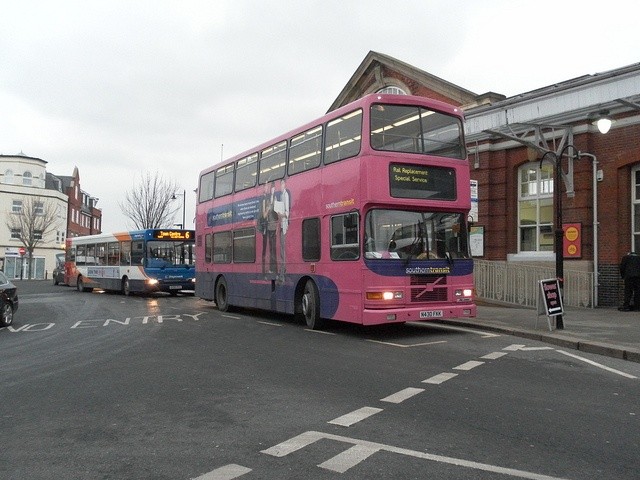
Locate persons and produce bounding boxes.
[266,183,278,275]
[276,179,289,281]
[258,186,269,273]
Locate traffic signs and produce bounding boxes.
[19,247,26,255]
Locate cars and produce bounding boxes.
[53,262,65,286]
[0,271,18,326]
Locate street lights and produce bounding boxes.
[171,190,185,229]
[174,224,182,229]
[540,145,582,330]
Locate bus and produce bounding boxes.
[64,229,195,296]
[195,93,477,330]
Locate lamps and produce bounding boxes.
[591,117,614,136]
